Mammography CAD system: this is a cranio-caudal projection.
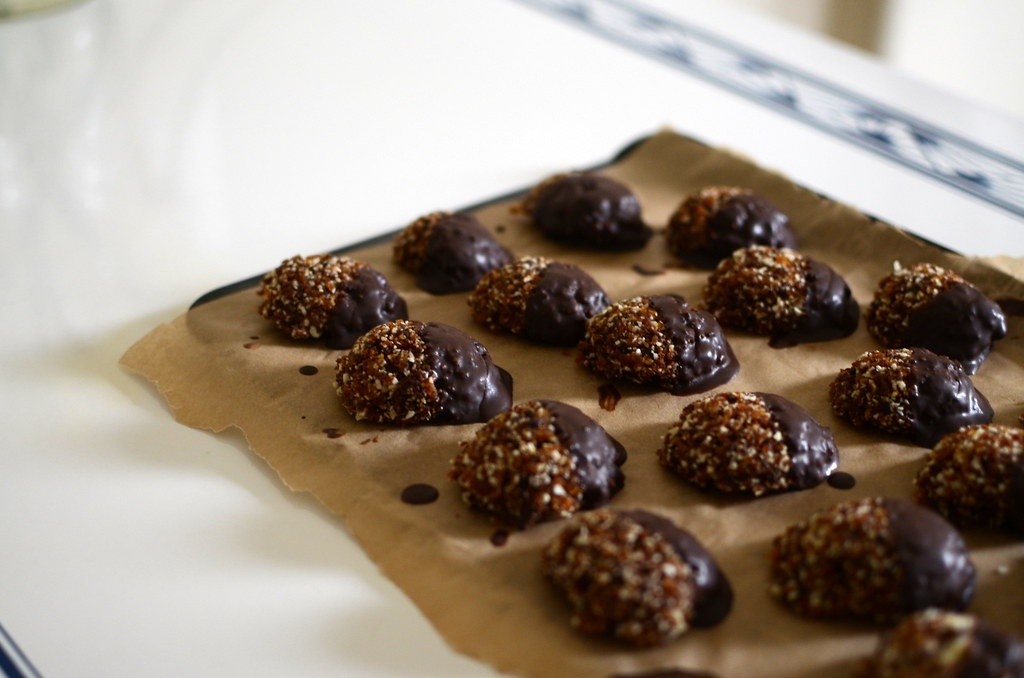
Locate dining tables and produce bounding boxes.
[0,0,1023,677]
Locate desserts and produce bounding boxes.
[256,174,1024,678]
